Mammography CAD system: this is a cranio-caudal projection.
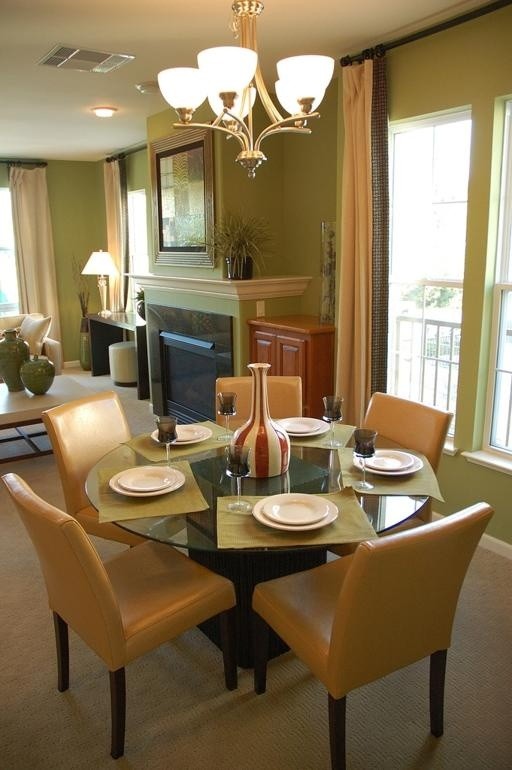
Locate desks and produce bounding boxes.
[87,312,149,401]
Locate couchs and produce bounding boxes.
[0,314,61,375]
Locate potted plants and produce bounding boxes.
[192,210,274,279]
[131,288,145,321]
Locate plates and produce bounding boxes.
[108,464,186,498]
[150,422,212,447]
[255,492,340,534]
[272,415,331,437]
[354,450,425,477]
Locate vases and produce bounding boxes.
[80,317,92,371]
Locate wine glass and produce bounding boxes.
[223,446,255,512]
[215,390,236,443]
[323,395,344,447]
[353,428,378,491]
[152,415,177,468]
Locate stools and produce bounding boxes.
[109,340,138,387]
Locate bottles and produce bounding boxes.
[226,361,292,477]
[0,329,55,396]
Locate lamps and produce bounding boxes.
[81,249,119,317]
[156,0,335,177]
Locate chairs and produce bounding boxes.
[216,376,303,417]
[252,501,494,769]
[328,393,454,560]
[40,389,188,549]
[1,472,236,759]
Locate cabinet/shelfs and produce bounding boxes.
[249,313,334,420]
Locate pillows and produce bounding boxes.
[19,316,52,356]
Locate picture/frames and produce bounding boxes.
[150,122,214,268]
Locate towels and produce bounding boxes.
[216,486,380,547]
[290,423,355,450]
[100,461,207,526]
[121,420,234,460]
[338,448,444,504]
